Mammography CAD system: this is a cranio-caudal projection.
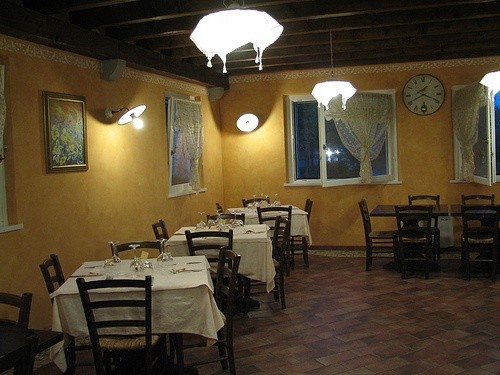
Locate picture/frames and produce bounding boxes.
[42,90,86,172]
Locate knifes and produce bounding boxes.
[69,275,103,277]
[242,232,264,234]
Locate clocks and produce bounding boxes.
[403,75,446,116]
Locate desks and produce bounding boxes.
[48,255,223,374]
[222,204,316,269]
[162,223,275,310]
[370,202,500,275]
[0,321,64,375]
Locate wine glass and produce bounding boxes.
[156,239,168,262]
[129,244,144,272]
[247,192,281,212]
[163,244,176,273]
[209,209,241,231]
[196,212,207,230]
[107,240,121,263]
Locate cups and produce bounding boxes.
[104,259,114,280]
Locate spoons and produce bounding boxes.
[246,230,265,233]
[176,269,205,272]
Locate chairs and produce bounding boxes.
[357,192,443,279]
[458,192,500,279]
[0,193,314,375]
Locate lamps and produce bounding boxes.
[311,32,357,112]
[479,67,500,96]
[105,103,147,129]
[190,1,283,72]
